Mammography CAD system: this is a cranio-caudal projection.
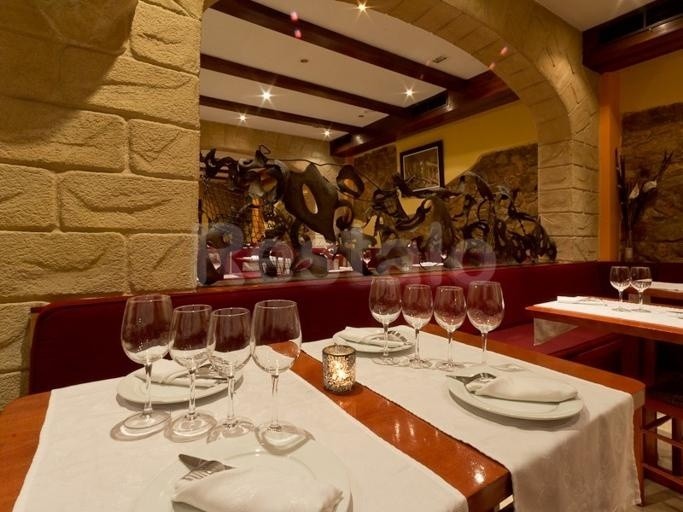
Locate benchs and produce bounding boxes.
[27,259,682,396]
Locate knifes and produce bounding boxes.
[445,374,476,386]
[178,453,233,474]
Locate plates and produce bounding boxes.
[170,445,352,511]
[332,326,416,354]
[448,363,584,421]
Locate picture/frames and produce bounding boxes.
[400,141,446,193]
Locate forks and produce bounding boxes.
[193,461,226,474]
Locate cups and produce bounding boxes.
[321,344,357,394]
[365,275,506,387]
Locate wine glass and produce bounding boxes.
[607,265,653,314]
[118,292,303,449]
